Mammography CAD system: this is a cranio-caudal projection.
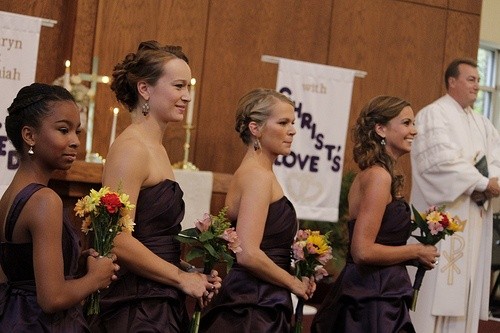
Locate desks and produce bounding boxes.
[51,161,231,323]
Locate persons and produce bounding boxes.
[311,96,440,333]
[83,40,222,333]
[0,82,121,333]
[201,87,316,333]
[405,57,500,333]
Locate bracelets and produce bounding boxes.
[481,191,488,199]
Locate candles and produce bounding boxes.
[108,108,119,147]
[64,60,71,90]
[186,78,196,126]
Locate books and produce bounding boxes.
[469,152,491,207]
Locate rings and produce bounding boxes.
[477,198,480,201]
[432,260,439,265]
[205,290,210,298]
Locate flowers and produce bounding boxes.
[175,205,242,333]
[407,203,467,310]
[76,182,137,317]
[291,228,335,333]
